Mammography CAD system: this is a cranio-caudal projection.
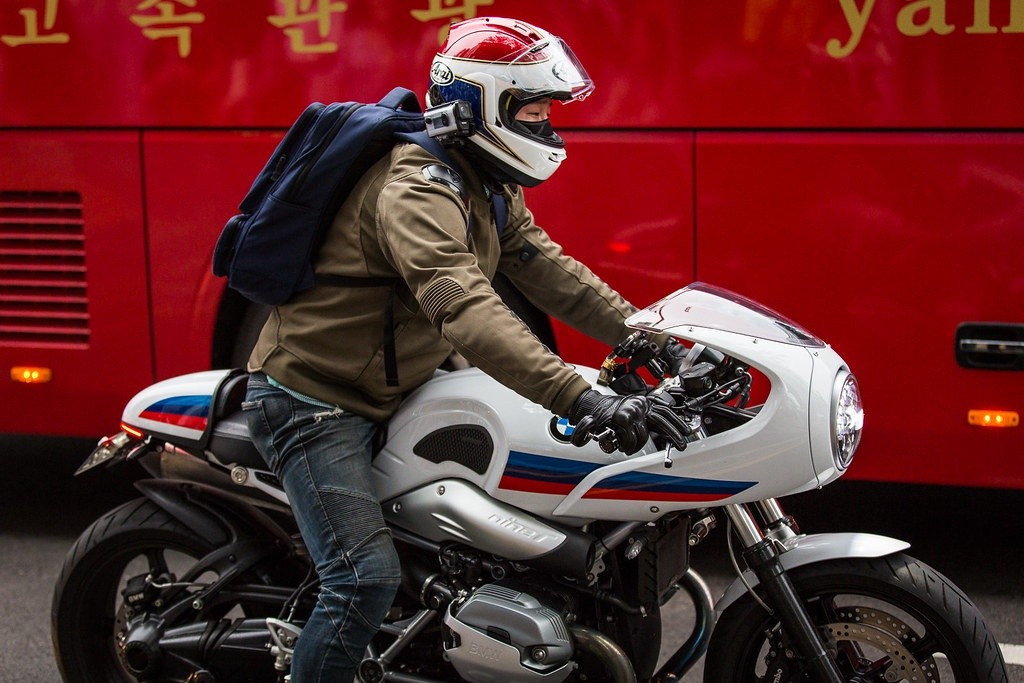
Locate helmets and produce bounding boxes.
[424,17,596,188]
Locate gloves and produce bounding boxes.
[569,388,692,456]
[659,336,692,378]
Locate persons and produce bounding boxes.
[242,16,690,683]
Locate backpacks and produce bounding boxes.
[212,85,508,308]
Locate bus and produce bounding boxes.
[1,0,1024,537]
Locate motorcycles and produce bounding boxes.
[50,280,1011,683]
